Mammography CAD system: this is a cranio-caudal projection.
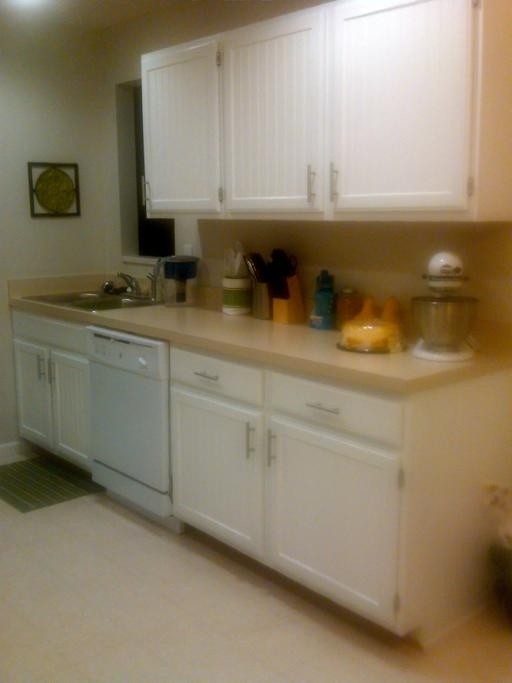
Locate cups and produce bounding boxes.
[163,253,199,307]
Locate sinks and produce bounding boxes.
[20,290,120,305]
[72,294,160,313]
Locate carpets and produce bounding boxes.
[0,457,92,514]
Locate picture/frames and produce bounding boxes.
[28,161,80,217]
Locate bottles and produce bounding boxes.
[222,274,253,316]
[309,269,336,329]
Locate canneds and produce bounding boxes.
[335,287,363,331]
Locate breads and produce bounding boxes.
[343,324,400,352]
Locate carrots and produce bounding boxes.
[360,295,375,321]
[383,296,400,322]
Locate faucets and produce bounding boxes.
[116,272,139,291]
[146,272,157,300]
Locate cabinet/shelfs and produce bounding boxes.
[170,347,512,656]
[10,310,91,470]
[226,0,512,222]
[140,33,226,220]
